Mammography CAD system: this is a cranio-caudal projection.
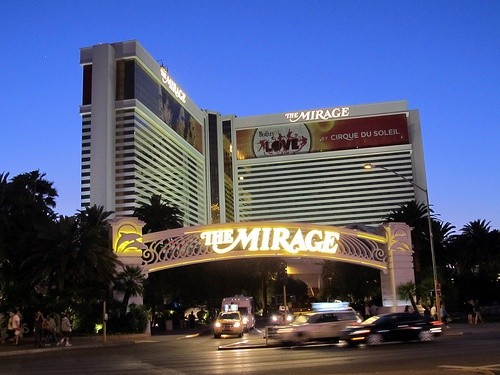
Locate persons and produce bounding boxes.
[364,303,377,318]
[0,310,22,346]
[33,309,72,348]
[404,305,410,312]
[467,300,474,324]
[431,305,436,320]
[189,311,196,331]
[475,299,484,324]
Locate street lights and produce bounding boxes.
[363,163,441,322]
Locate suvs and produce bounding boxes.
[277,310,359,347]
[213,312,244,338]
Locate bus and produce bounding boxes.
[221,297,256,334]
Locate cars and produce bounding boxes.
[339,313,444,348]
[270,309,293,324]
[348,309,363,323]
